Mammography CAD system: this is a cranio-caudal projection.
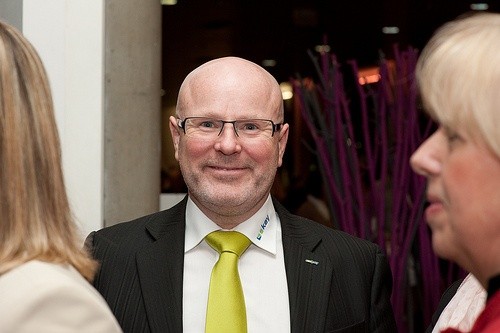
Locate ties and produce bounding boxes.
[206,231,252,333]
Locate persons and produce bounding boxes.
[409,13,500,333]
[0,20,123,333]
[79,56,397,333]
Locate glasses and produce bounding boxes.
[176,116,283,142]
